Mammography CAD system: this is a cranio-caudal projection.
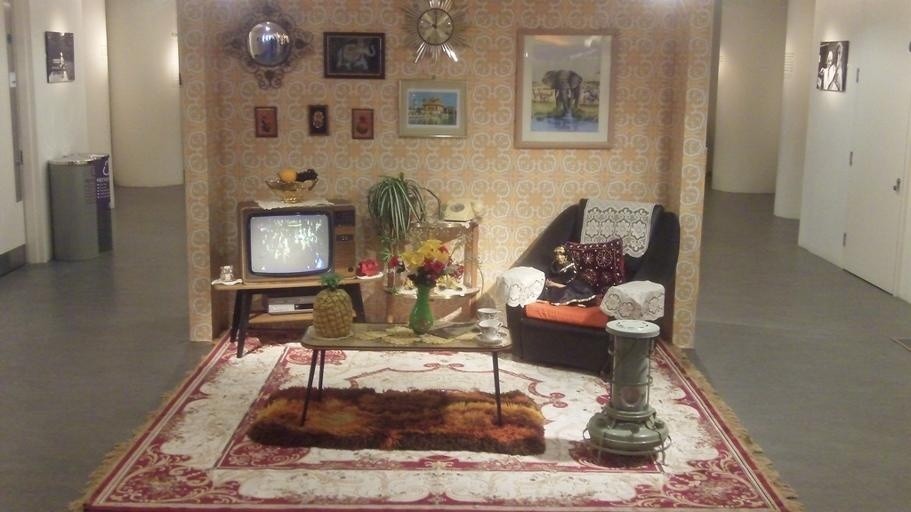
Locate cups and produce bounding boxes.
[478,319,503,340]
[477,308,504,319]
[219,265,234,282]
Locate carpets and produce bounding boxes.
[69,324,807,512]
[246,386,546,455]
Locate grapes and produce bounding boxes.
[297,169,317,182]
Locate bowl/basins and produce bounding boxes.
[264,176,319,205]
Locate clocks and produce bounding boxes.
[399,0,471,64]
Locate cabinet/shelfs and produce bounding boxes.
[382,221,481,325]
[213,277,384,358]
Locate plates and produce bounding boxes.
[475,332,506,345]
[356,272,383,280]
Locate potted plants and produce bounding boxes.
[367,173,443,262]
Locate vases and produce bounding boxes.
[408,284,437,334]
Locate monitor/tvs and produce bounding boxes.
[239,197,358,286]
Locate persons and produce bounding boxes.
[817,42,843,91]
[537,246,596,308]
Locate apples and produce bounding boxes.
[358,259,379,276]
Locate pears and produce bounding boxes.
[278,170,297,182]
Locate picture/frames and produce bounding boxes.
[398,77,469,140]
[514,28,619,149]
[352,109,374,140]
[255,107,278,137]
[309,105,328,136]
[323,31,386,79]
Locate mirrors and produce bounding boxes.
[222,0,314,89]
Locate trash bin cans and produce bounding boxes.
[48,152,113,261]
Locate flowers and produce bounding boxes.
[388,239,465,291]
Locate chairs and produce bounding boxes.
[503,199,680,373]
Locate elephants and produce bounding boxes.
[542,69,584,117]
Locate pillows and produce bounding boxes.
[555,238,624,305]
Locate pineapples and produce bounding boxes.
[312,270,352,338]
